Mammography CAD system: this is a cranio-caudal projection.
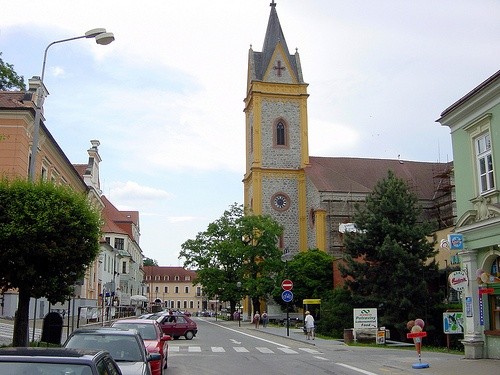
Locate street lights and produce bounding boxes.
[29,28,115,184]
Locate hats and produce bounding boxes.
[305,311,310,314]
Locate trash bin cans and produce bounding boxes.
[343,328,353,343]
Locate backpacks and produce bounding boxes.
[263,314,267,318]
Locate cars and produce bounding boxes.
[62,327,162,374]
[155,314,199,339]
[137,309,169,320]
[180,309,192,317]
[0,346,122,375]
[110,319,172,375]
[204,310,220,317]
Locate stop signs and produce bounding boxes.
[282,279,294,291]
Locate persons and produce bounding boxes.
[254,311,260,328]
[305,311,315,342]
[163,312,176,340]
[261,310,268,328]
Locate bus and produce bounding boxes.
[226,305,244,317]
